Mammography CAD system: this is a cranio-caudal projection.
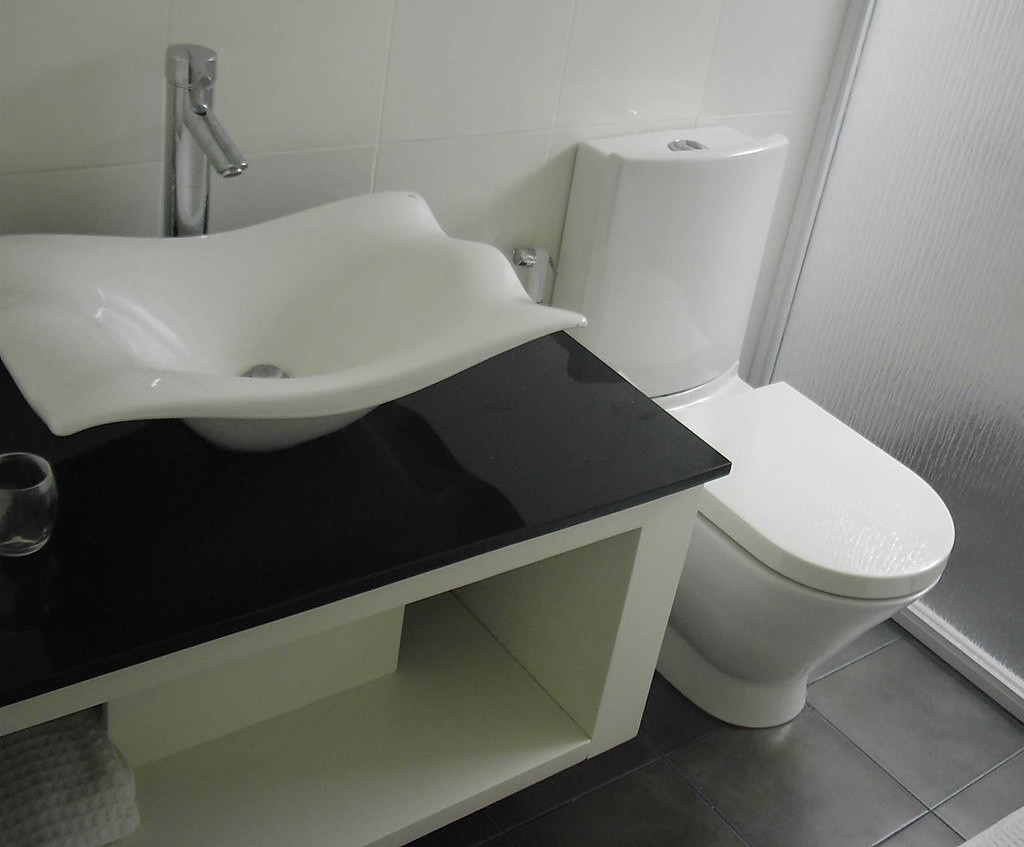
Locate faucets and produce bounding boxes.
[157,41,249,237]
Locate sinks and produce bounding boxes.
[1,191,592,450]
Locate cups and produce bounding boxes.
[0,452,57,555]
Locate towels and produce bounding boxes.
[0,721,142,847]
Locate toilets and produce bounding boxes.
[544,123,956,730]
